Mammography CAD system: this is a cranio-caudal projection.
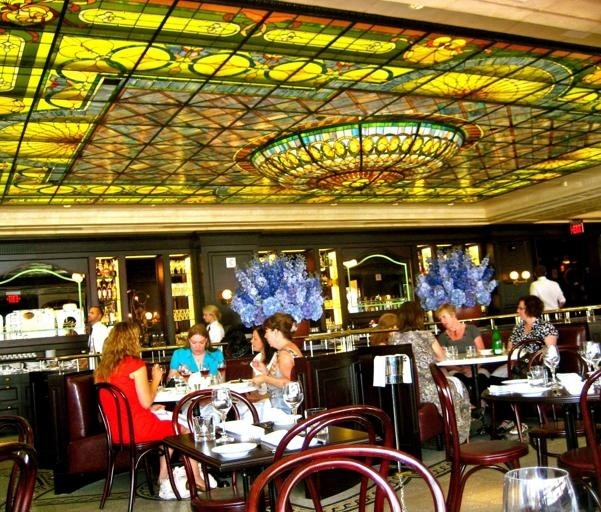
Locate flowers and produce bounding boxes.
[230,255,323,325]
[413,248,495,311]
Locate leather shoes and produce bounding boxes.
[187,480,215,492]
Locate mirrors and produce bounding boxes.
[347,255,410,309]
[0,269,80,339]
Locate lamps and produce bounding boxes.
[249,121,464,193]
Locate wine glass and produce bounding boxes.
[282,379,305,430]
[501,465,580,512]
[211,387,235,443]
[159,360,227,386]
[542,340,601,386]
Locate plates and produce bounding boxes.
[211,442,258,459]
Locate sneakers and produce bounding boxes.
[497,420,528,434]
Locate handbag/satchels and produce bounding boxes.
[159,466,217,500]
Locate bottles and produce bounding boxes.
[172,267,187,283]
[327,321,356,333]
[491,325,503,356]
[96,263,117,300]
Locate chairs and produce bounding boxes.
[0,442,36,511]
[427,363,530,512]
[245,445,444,511]
[487,337,546,442]
[0,414,32,447]
[266,403,393,511]
[172,390,260,511]
[91,382,181,511]
[533,350,588,466]
[556,371,601,512]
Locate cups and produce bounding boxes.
[0,352,38,360]
[447,344,459,356]
[304,407,329,437]
[192,415,217,443]
[544,309,596,324]
[526,365,549,386]
[464,345,477,358]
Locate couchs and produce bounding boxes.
[415,324,586,462]
[52,357,318,501]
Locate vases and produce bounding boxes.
[431,303,482,322]
[291,320,310,341]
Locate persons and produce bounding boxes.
[94,323,206,493]
[529,257,567,319]
[498,295,560,434]
[202,304,226,352]
[249,312,303,415]
[87,305,110,370]
[369,303,485,445]
[167,324,226,383]
